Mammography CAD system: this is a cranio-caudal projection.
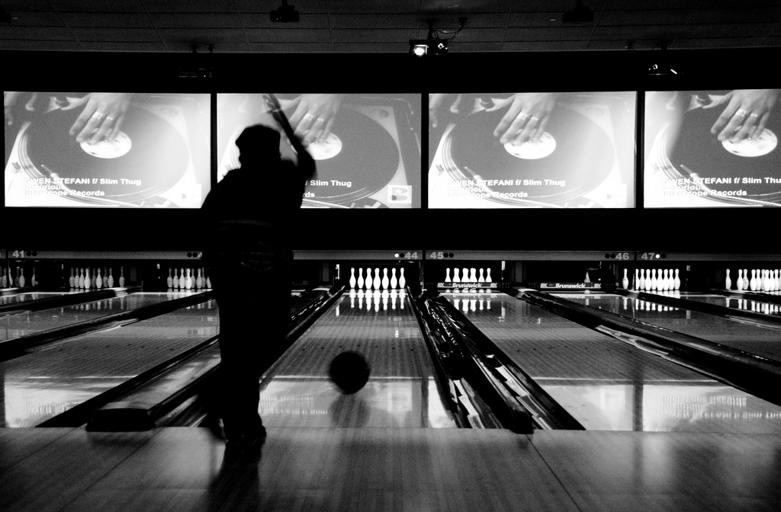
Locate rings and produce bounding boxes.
[318,117,326,124]
[107,116,115,121]
[304,111,316,121]
[751,113,760,119]
[517,112,530,121]
[93,111,105,119]
[737,109,749,117]
[531,116,540,122]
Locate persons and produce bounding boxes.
[429,93,560,144]
[195,92,316,445]
[702,88,779,144]
[4,91,131,145]
[278,93,344,148]
